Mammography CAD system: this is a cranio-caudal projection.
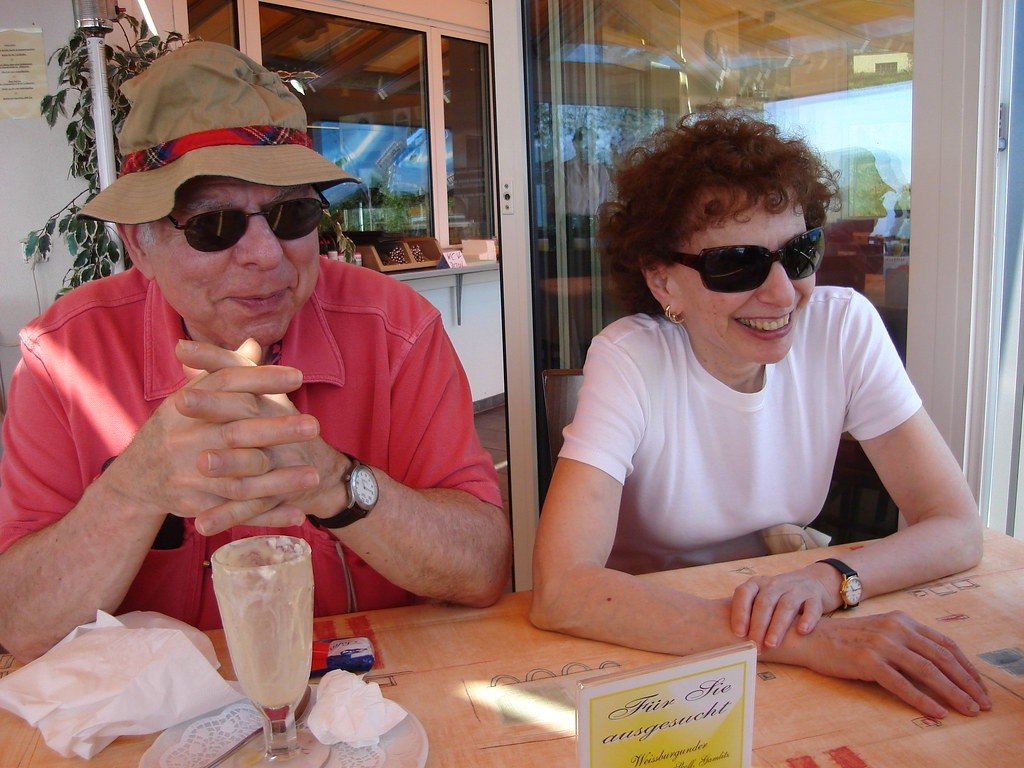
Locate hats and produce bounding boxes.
[74,40,363,223]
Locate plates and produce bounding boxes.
[138,683,428,768]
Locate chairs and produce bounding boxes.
[534,369,585,477]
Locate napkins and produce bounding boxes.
[305,667,413,750]
[0,608,248,760]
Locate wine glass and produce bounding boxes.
[210,535,331,768]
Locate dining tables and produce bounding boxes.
[0,523,1024,768]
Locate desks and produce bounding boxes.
[540,275,591,366]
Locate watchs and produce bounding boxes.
[305,449,378,530]
[815,558,863,610]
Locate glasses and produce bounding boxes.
[167,184,331,253]
[668,228,825,293]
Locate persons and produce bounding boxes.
[562,124,612,246]
[529,108,994,721]
[1,46,511,665]
